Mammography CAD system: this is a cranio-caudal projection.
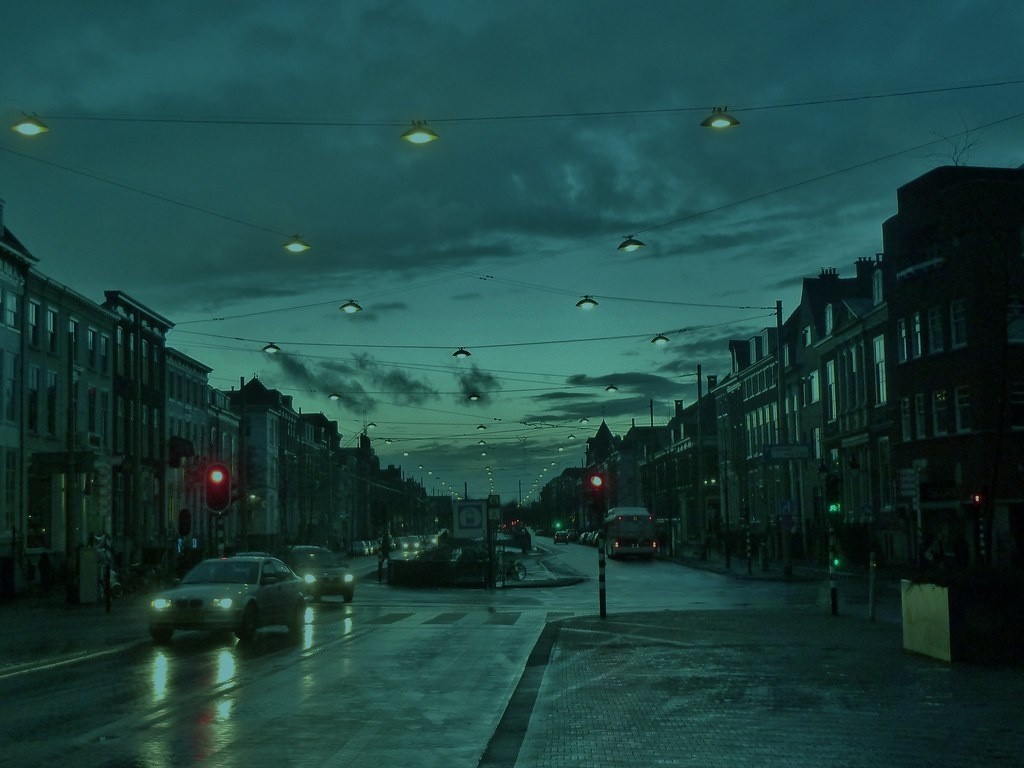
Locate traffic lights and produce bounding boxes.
[590,471,605,493]
[826,487,842,567]
[206,464,232,515]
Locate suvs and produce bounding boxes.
[289,545,356,602]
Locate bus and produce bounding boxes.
[603,506,658,561]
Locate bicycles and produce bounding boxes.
[497,557,527,581]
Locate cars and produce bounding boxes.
[347,535,437,556]
[535,528,600,547]
[237,551,271,557]
[148,556,308,641]
